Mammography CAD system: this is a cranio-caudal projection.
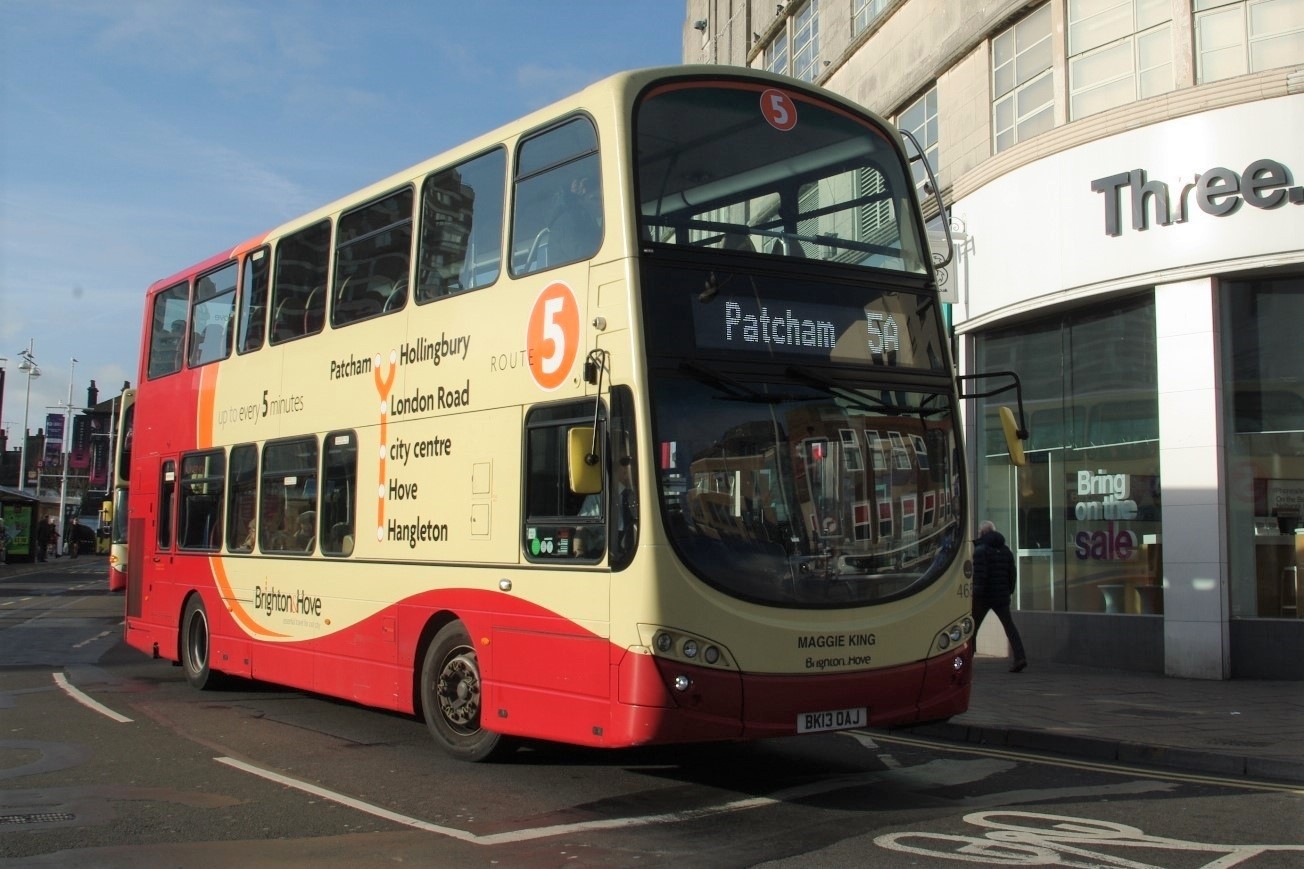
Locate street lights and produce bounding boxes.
[18,337,42,492]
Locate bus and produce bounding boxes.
[123,68,1030,769]
[99,382,136,593]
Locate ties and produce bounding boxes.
[622,489,633,561]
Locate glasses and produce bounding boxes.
[618,457,636,467]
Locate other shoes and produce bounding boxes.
[70,556,76,558]
[38,559,47,563]
[1009,661,1027,673]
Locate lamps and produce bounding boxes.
[694,19,707,31]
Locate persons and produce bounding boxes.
[66,518,81,559]
[573,452,638,559]
[237,510,316,552]
[547,171,603,266]
[0,518,12,551]
[151,318,186,377]
[970,520,1027,674]
[37,515,61,563]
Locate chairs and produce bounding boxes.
[160,483,352,553]
[174,205,806,374]
